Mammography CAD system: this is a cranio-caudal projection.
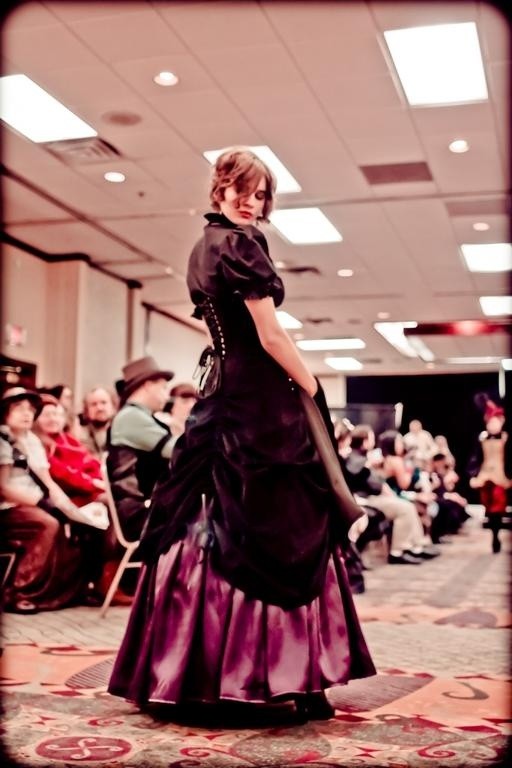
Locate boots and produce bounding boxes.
[100,561,132,606]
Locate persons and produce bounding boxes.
[465,393,511,552]
[106,358,201,551]
[329,417,470,566]
[107,148,371,729]
[2,383,139,613]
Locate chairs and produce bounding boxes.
[94,448,151,620]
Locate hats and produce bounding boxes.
[0,386,59,421]
[484,400,504,421]
[122,356,174,392]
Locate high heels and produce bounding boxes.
[294,690,334,725]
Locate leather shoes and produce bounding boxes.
[78,595,101,606]
[388,550,440,564]
